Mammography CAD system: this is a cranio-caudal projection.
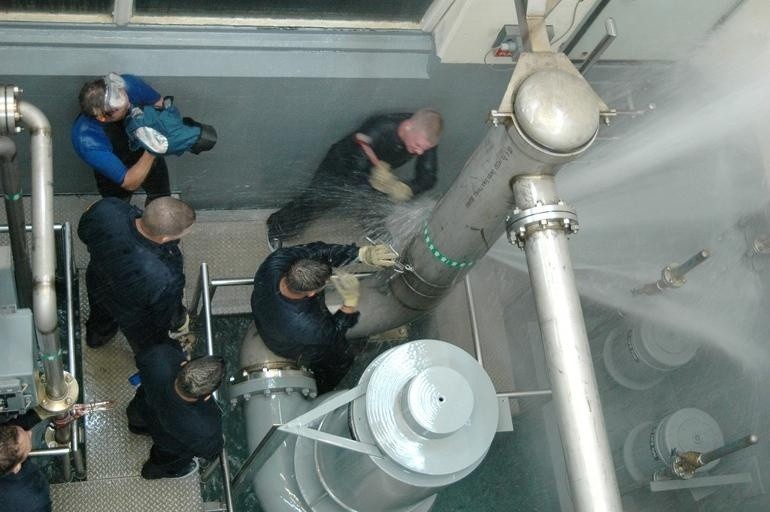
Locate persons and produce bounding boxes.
[251,241,397,394]
[0,409,52,510]
[266,107,445,251]
[77,198,196,358]
[71,74,171,207]
[126,343,227,478]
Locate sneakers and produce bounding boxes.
[267,229,282,252]
[165,460,198,478]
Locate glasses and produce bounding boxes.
[104,72,126,114]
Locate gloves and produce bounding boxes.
[371,160,412,208]
[359,245,396,267]
[331,267,360,306]
